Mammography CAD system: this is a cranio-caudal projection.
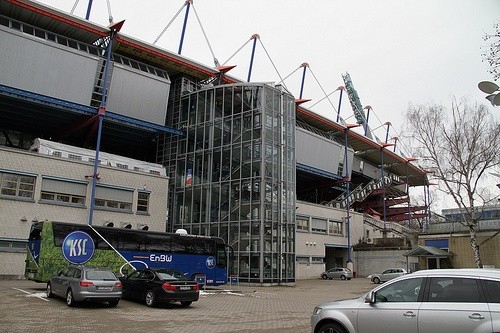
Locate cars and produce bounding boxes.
[371,268,408,284]
[121,269,199,307]
[47,265,122,307]
[320,268,353,280]
[310,268,500,333]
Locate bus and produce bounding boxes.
[23,220,235,283]
